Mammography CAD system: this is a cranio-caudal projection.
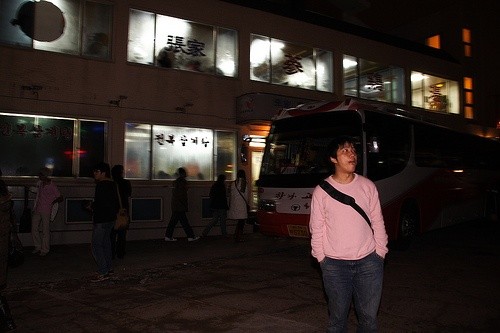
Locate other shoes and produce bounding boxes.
[31,248,40,254]
[40,252,51,256]
[165,237,178,241]
[187,236,200,241]
[7,234,24,269]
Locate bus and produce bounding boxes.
[237,99,499,251]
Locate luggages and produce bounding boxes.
[91,271,113,282]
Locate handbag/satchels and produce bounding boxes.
[114,207,130,230]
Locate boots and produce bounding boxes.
[0,296,17,333]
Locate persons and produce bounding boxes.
[227,168,251,240]
[309,134,389,333]
[202,172,229,238]
[0,169,19,253]
[85,163,120,282]
[29,166,62,254]
[164,167,201,241]
